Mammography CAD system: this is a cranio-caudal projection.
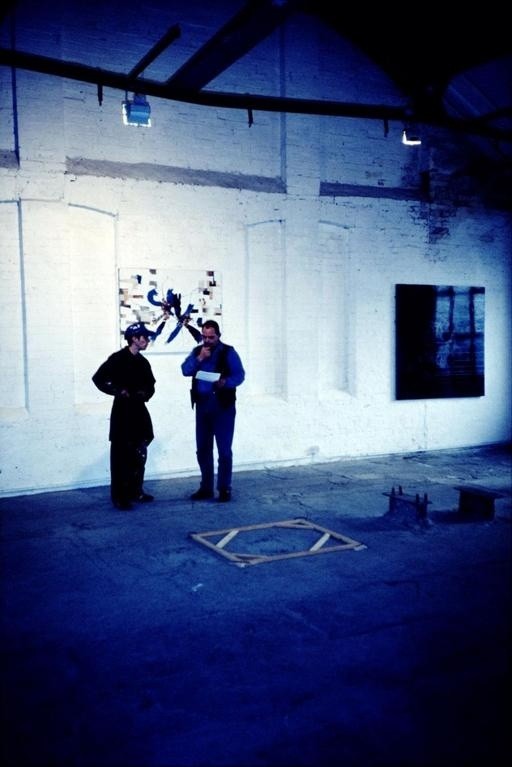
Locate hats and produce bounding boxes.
[124,322,155,340]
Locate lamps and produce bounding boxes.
[120,91,153,127]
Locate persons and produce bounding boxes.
[181,319,245,502]
[91,320,156,511]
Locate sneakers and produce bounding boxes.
[134,492,156,502]
[217,491,231,502]
[191,487,214,501]
